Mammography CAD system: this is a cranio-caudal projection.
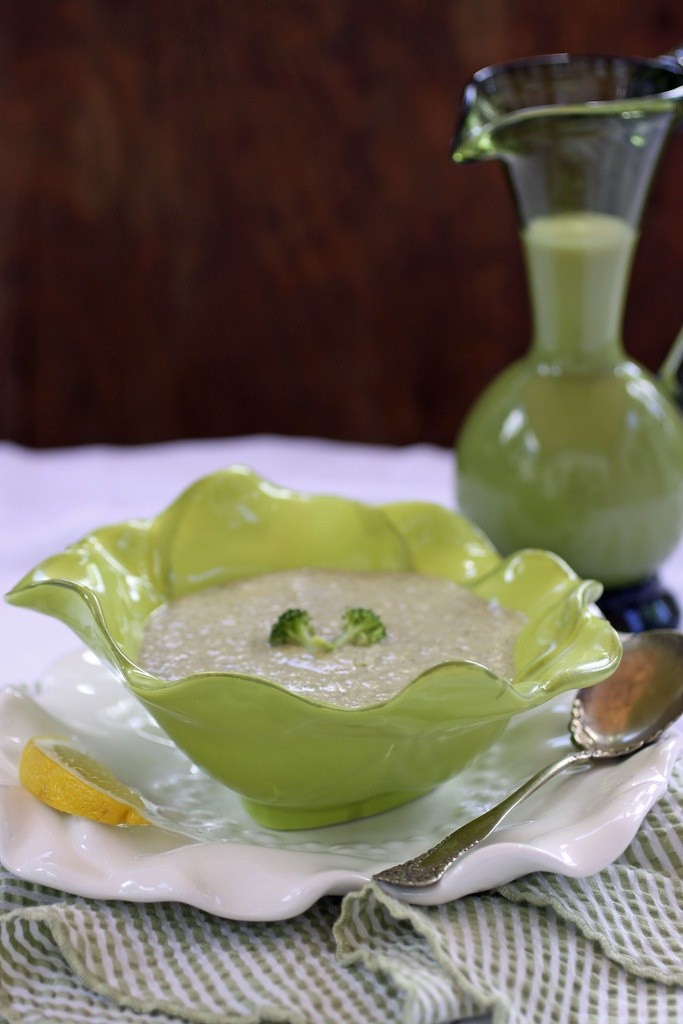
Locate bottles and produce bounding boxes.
[451,50,680,591]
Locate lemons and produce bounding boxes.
[20,735,150,828]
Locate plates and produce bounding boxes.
[1,437,681,923]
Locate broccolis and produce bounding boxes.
[333,608,384,649]
[270,609,330,655]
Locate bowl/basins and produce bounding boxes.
[4,465,625,830]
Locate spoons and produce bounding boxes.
[374,628,683,887]
